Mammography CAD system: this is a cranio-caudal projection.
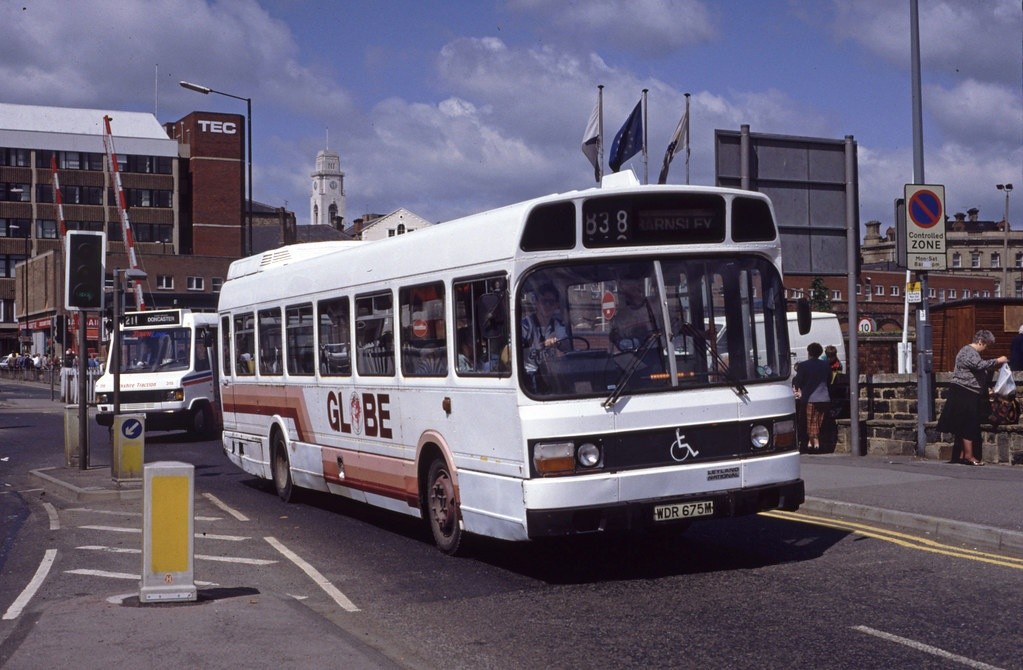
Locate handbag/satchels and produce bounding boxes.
[994,363,1017,397]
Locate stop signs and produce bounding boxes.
[412,319,429,338]
[601,290,616,321]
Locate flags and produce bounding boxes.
[582,92,599,182]
[658,111,686,185]
[608,100,644,172]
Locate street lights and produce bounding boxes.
[996,183,1014,298]
[179,79,255,256]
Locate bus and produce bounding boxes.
[212,168,806,561]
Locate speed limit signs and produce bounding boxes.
[858,318,872,333]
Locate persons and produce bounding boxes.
[456,326,485,372]
[608,275,717,369]
[377,331,445,373]
[574,303,602,328]
[501,283,571,371]
[822,345,842,372]
[272,341,283,373]
[6,348,103,383]
[1009,324,1023,371]
[936,330,1009,466]
[236,348,255,374]
[793,342,833,454]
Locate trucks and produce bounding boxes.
[91,307,224,443]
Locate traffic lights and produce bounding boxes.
[64,228,107,311]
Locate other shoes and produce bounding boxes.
[964,458,985,466]
[807,447,814,453]
[959,457,964,463]
[813,447,820,453]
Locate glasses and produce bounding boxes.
[541,299,555,305]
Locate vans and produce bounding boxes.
[663,309,849,420]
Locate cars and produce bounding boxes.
[321,342,351,374]
